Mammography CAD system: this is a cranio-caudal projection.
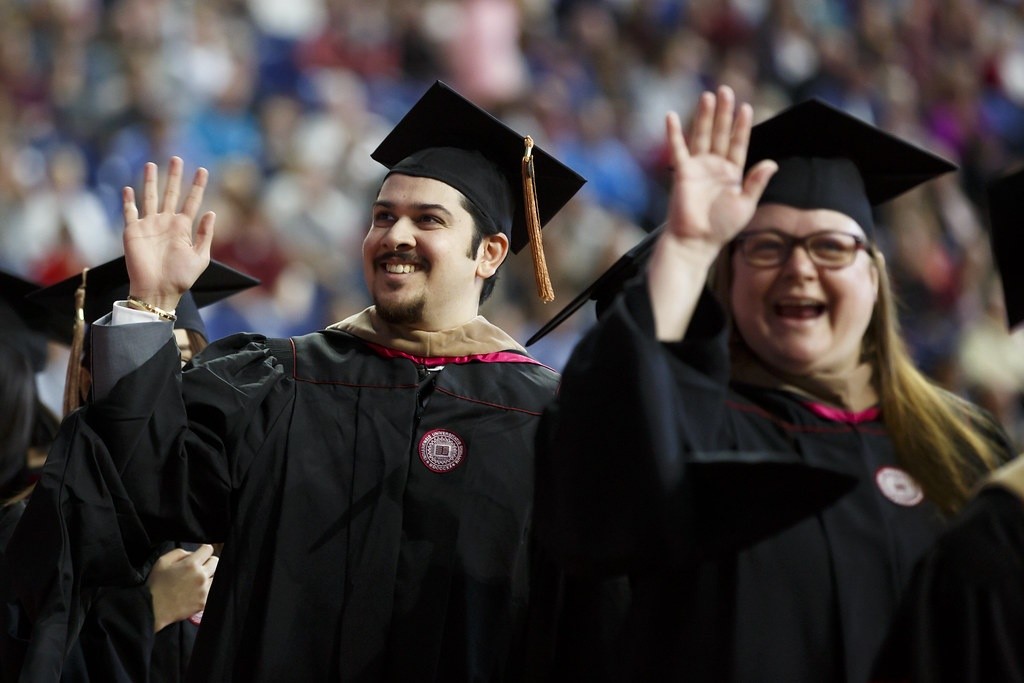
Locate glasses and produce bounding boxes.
[726,228,875,269]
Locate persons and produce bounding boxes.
[536,81,1020,683]
[68,81,563,683]
[37,258,260,683]
[0,1,1022,397]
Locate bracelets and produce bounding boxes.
[125,293,179,322]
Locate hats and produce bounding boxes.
[370,80,587,256]
[732,99,956,239]
[23,254,262,358]
[527,220,673,350]
